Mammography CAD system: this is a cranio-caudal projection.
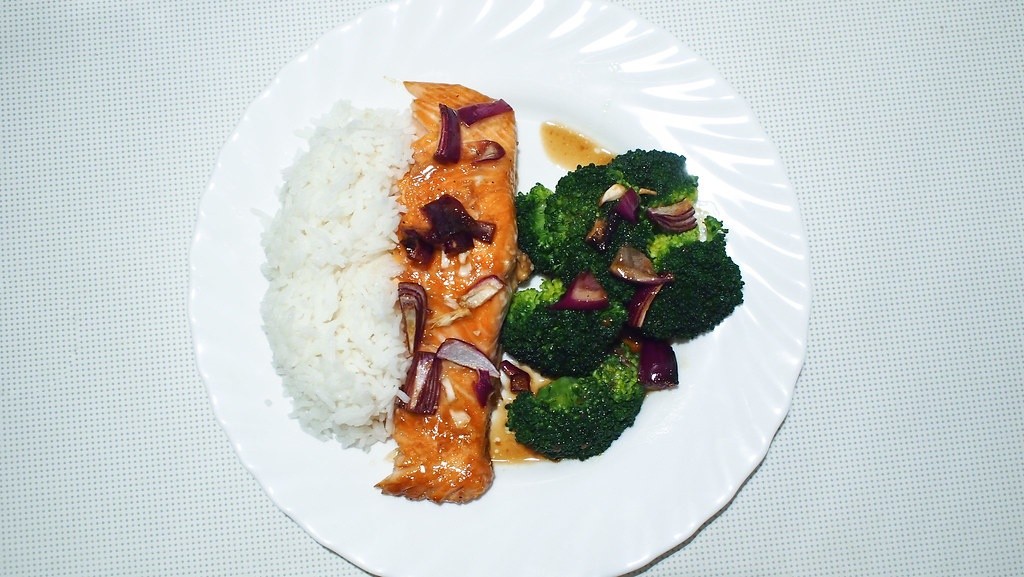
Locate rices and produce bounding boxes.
[260,105,426,453]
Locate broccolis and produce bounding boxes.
[503,148,745,462]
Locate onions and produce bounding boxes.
[395,99,698,417]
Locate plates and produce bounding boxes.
[189,1,812,577]
